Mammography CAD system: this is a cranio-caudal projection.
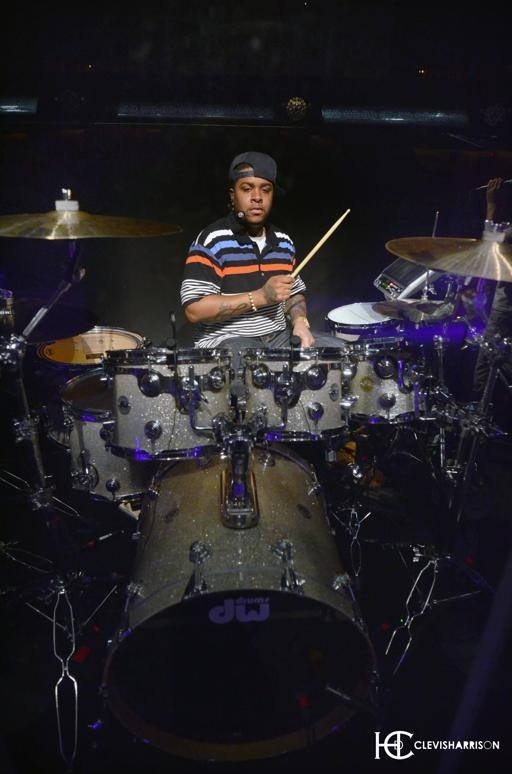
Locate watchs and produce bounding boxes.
[291,316,311,328]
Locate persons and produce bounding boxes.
[178,150,315,349]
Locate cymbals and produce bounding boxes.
[0,297,99,342]
[0,208,184,240]
[372,300,455,322]
[384,233,512,285]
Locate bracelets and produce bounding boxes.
[247,290,256,312]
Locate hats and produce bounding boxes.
[229,150,279,189]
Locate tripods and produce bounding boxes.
[2,382,128,774]
[356,393,487,682]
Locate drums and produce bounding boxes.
[346,337,438,431]
[325,303,413,360]
[33,322,146,415]
[238,343,354,446]
[106,347,235,463]
[57,372,149,505]
[97,443,379,760]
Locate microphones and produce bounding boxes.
[229,201,247,224]
